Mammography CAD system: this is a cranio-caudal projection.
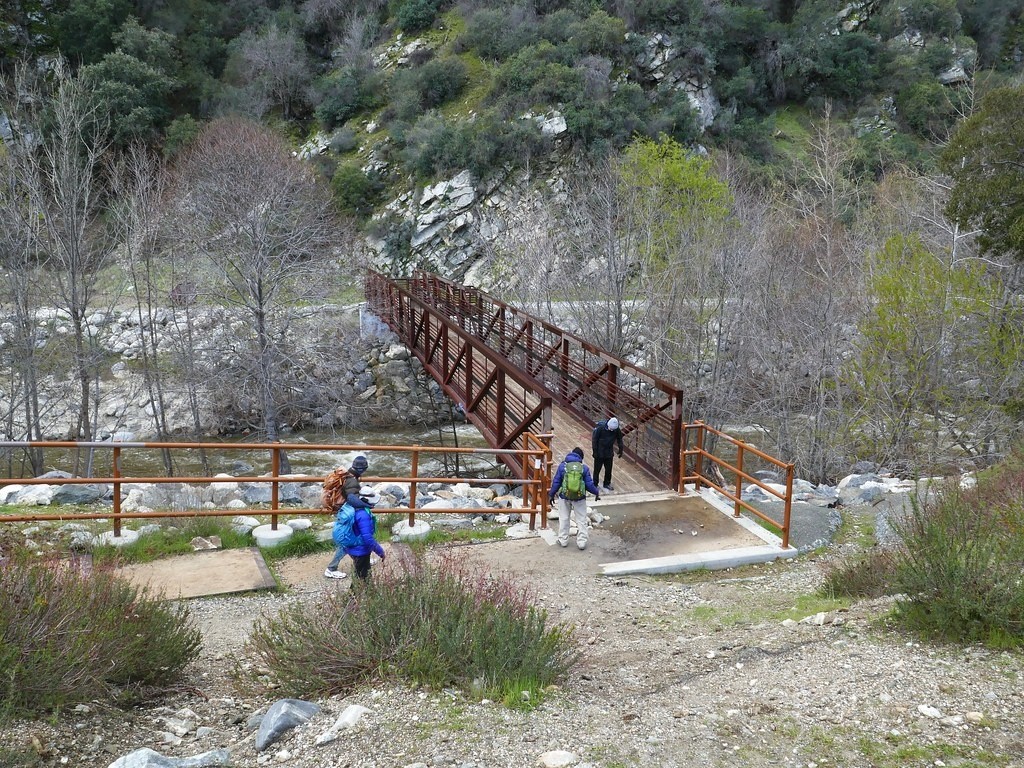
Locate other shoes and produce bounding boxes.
[603,484,614,490]
[577,545,584,549]
[556,540,567,546]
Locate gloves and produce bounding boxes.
[594,451,598,459]
[617,448,623,458]
[595,493,601,501]
[549,496,555,507]
[380,551,385,562]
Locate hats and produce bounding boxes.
[572,447,584,459]
[358,486,381,504]
[352,456,368,472]
[607,417,618,429]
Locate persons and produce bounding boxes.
[548,447,602,550]
[592,417,624,490]
[324,456,386,599]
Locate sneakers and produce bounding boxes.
[324,568,347,578]
[370,558,377,565]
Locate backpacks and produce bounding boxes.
[558,462,586,501]
[332,502,364,561]
[319,467,354,515]
[593,420,606,435]
